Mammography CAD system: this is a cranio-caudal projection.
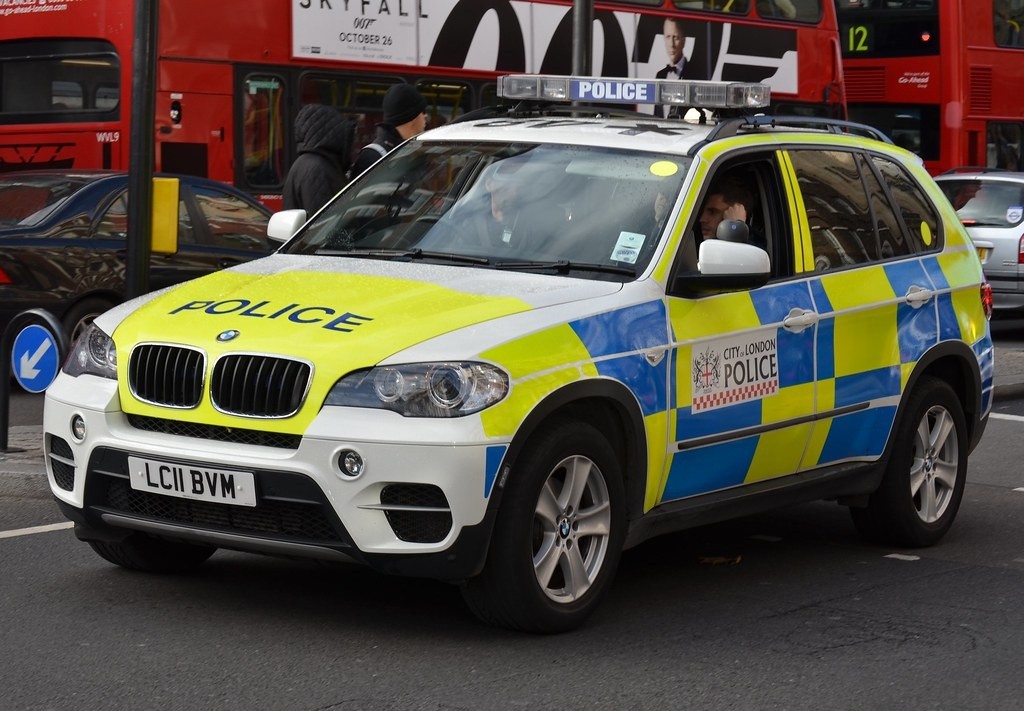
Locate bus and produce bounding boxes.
[833,0,1024,177]
[0,0,851,279]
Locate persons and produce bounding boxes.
[453,164,569,250]
[638,186,676,248]
[699,186,766,250]
[656,16,707,80]
[346,83,429,182]
[283,103,350,221]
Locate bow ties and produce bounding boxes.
[666,63,678,74]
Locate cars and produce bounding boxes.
[0,170,297,356]
[932,166,1024,306]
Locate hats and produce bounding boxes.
[383,82,425,126]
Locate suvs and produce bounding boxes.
[41,113,995,635]
